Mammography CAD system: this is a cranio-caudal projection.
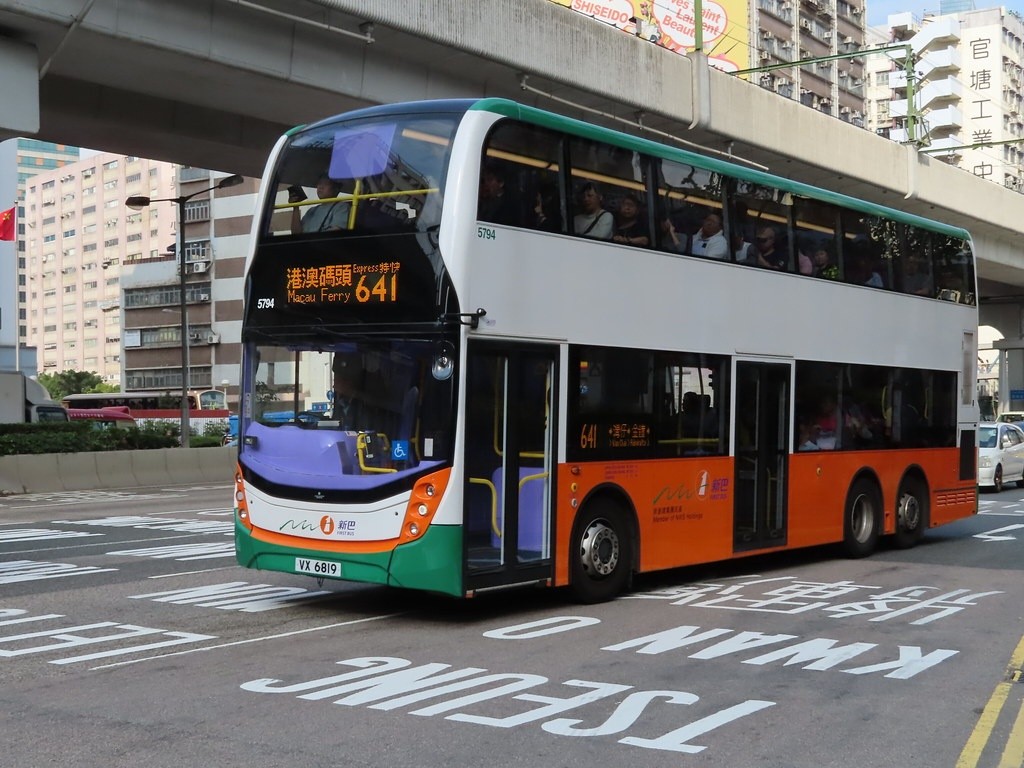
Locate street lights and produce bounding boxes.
[124,174,245,448]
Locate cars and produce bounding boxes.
[994,411,1024,423]
[978,422,1024,492]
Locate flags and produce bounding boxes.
[0,207,15,241]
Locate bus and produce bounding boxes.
[66,405,138,429]
[59,388,231,437]
[231,96,982,604]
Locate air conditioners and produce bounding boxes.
[819,61,829,68]
[1002,70,1024,194]
[207,336,221,344]
[193,262,206,273]
[852,8,861,14]
[854,78,862,85]
[844,37,854,43]
[198,294,209,300]
[802,51,813,58]
[839,71,848,78]
[859,46,867,51]
[763,32,775,39]
[782,0,832,38]
[781,40,792,49]
[779,77,788,86]
[799,87,894,134]
[762,51,771,59]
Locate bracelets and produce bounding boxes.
[627,237,632,242]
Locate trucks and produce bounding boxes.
[1,370,70,423]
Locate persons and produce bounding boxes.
[108,399,158,409]
[813,248,840,281]
[288,173,350,232]
[613,194,651,246]
[754,227,785,269]
[797,384,922,450]
[332,357,365,430]
[480,168,933,297]
[222,428,233,445]
[676,393,701,433]
[574,181,612,239]
[692,211,728,258]
